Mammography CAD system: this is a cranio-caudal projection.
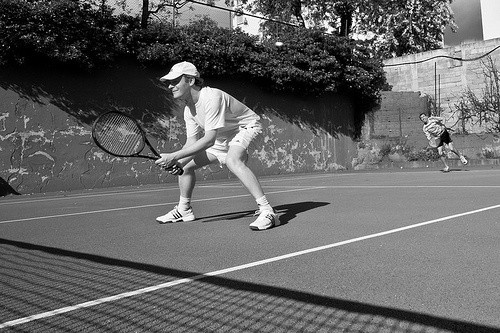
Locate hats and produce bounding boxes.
[160,61,200,82]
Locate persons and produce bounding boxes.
[419,113,468,172]
[155,60,281,231]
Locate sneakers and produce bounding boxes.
[249,210,281,231]
[155,204,195,224]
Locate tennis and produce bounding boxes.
[426,164,429,167]
[401,166,403,169]
